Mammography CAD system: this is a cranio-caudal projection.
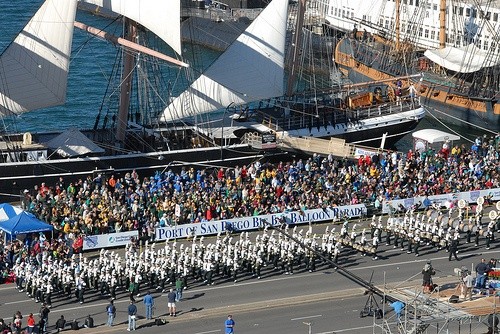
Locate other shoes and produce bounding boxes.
[28,294,30,296]
[184,287,187,289]
[21,289,23,291]
[137,294,140,296]
[127,329,130,331]
[19,290,22,292]
[26,292,29,294]
[173,314,175,316]
[169,314,172,316]
[36,300,38,302]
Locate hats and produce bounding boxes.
[437,203,441,208]
[234,223,339,261]
[344,210,492,236]
[16,249,123,284]
[126,232,233,273]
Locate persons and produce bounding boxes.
[362,28,368,43]
[394,79,418,107]
[373,86,382,105]
[353,25,358,39]
[281,102,292,117]
[0,131,500,334]
[341,104,350,114]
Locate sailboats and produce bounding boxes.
[333,0,500,135]
[0,0,428,203]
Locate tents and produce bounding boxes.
[0,210,54,246]
[44,127,105,157]
[423,42,500,73]
[0,203,28,222]
